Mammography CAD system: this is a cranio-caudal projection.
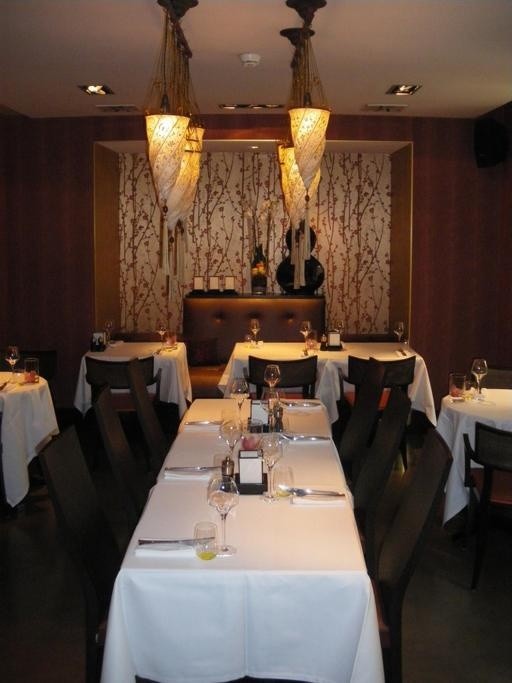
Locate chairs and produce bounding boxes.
[249,355,318,400]
[337,354,453,681]
[463,421,512,590]
[16,349,45,373]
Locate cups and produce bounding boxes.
[25,358,39,383]
[193,520,222,561]
[166,335,177,349]
[449,372,467,404]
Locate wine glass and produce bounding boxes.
[208,365,283,557]
[5,346,20,377]
[158,323,166,349]
[104,320,113,346]
[471,359,488,397]
[249,318,261,343]
[299,320,313,352]
[393,322,405,353]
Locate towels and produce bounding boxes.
[288,434,331,446]
[164,467,212,480]
[287,405,322,410]
[134,540,192,555]
[184,423,220,430]
[291,493,342,504]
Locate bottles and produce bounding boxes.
[251,243,267,297]
[320,333,328,351]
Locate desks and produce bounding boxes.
[182,294,327,368]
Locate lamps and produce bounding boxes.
[143,0,207,286]
[277,2,331,289]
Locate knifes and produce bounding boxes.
[138,400,345,546]
